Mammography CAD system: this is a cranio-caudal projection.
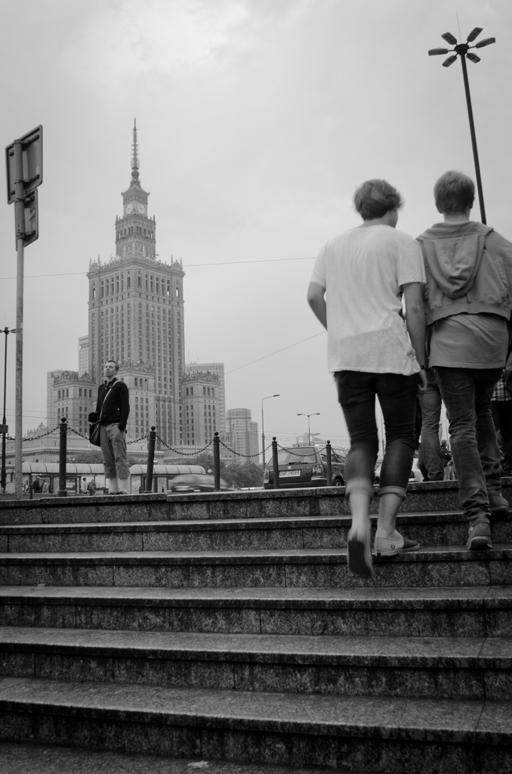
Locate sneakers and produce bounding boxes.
[467,522,493,550]
[488,496,510,513]
[375,535,421,557]
[346,529,374,578]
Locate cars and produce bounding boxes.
[261,444,348,488]
[169,473,237,491]
[374,455,423,485]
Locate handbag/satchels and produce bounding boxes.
[87,424,99,447]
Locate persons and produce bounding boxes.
[413,169,511,550]
[415,368,444,482]
[88,359,133,495]
[304,179,431,578]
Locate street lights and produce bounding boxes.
[1,327,21,493]
[260,392,280,470]
[297,411,321,445]
[428,19,498,225]
